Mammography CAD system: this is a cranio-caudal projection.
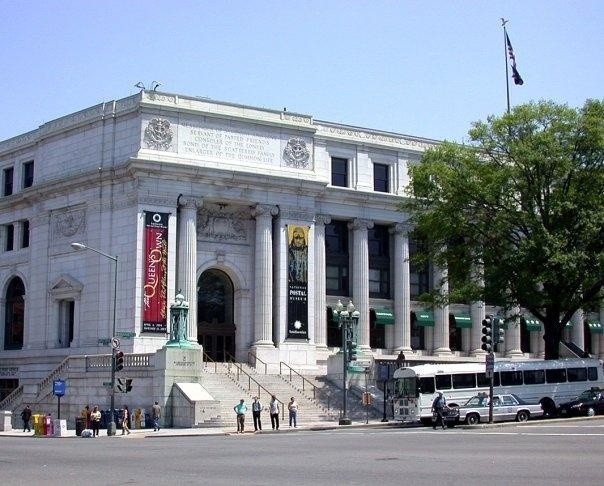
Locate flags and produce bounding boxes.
[503,27,523,82]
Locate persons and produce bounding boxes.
[151,401,160,432]
[121,404,130,436]
[252,396,261,429]
[91,406,99,436]
[233,400,246,435]
[18,406,31,432]
[290,227,308,283]
[431,391,450,430]
[83,406,90,436]
[268,395,279,431]
[287,397,298,427]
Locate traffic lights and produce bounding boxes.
[115,351,123,372]
[480,316,493,353]
[126,379,133,393]
[497,319,506,345]
[117,378,125,392]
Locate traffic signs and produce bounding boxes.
[52,379,66,396]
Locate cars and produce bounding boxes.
[555,389,603,418]
[443,393,543,426]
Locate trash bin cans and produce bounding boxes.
[76,417,86,436]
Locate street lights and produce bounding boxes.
[335,299,362,425]
[70,242,119,437]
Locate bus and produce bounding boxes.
[392,357,604,422]
